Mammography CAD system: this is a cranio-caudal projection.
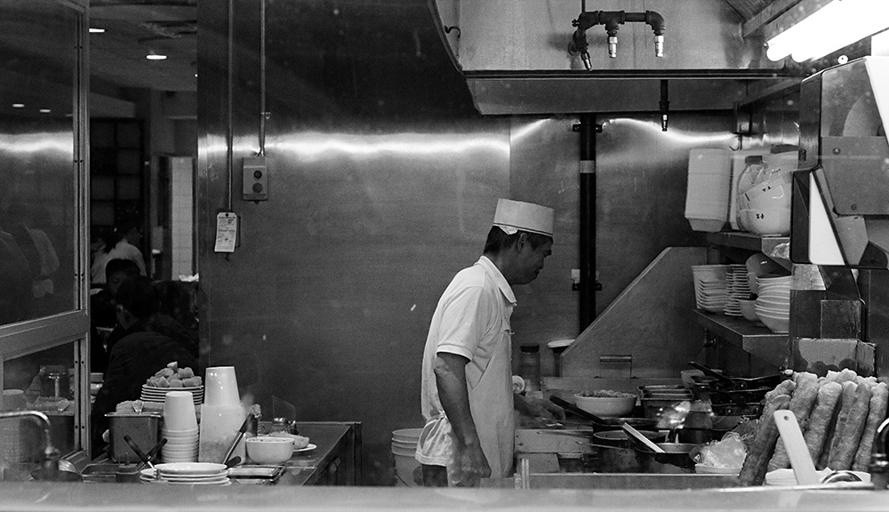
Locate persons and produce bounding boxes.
[90,213,148,291]
[89,275,193,432]
[89,256,142,317]
[0,198,76,325]
[416,196,567,491]
[154,279,203,346]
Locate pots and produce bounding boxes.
[530,416,667,434]
[685,360,778,416]
[634,442,709,471]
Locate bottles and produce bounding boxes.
[519,343,540,384]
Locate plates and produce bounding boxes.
[698,279,726,309]
[140,462,231,486]
[229,464,286,482]
[725,264,750,316]
[692,264,724,311]
[685,150,729,230]
[139,385,204,403]
[294,444,317,452]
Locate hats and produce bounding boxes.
[493,198,555,237]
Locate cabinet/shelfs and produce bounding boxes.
[685,79,793,375]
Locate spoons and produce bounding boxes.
[123,434,154,467]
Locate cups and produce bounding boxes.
[199,366,246,465]
[162,391,198,462]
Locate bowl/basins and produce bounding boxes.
[755,275,791,335]
[573,391,636,414]
[736,296,755,319]
[741,178,792,228]
[246,437,294,465]
[639,383,693,416]
[593,430,664,448]
[104,410,160,461]
